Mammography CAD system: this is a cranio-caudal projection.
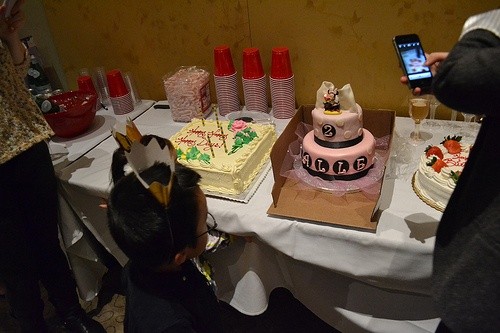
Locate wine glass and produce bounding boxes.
[458,111,475,138]
[443,107,463,129]
[407,92,430,140]
[423,94,442,129]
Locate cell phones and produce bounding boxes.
[392,33,434,90]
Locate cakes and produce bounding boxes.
[300,80,375,180]
[414,135,474,208]
[167,119,277,195]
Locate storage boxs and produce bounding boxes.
[161,66,213,123]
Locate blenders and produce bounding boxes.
[18,35,53,95]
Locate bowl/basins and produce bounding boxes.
[37,91,97,140]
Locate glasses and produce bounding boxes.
[179,212,218,243]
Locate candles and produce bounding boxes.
[197,102,228,158]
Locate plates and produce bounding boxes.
[224,110,269,122]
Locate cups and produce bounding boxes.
[160,64,212,123]
[213,45,241,117]
[76,66,141,116]
[241,47,268,114]
[269,47,296,119]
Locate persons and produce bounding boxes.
[108,116,243,301]
[0,0,123,333]
[399,9,500,333]
[0,0,106,333]
[105,137,223,333]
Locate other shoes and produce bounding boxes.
[60,308,107,333]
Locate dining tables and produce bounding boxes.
[44,98,481,333]
